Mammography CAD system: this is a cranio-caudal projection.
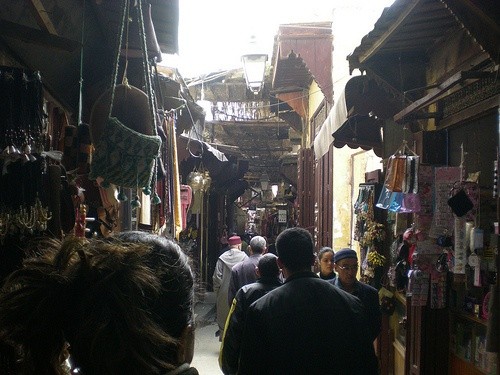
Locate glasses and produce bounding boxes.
[339,265,358,271]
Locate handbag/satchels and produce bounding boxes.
[88,118,162,196]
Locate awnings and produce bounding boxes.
[180,134,228,162]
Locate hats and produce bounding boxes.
[228,235,242,244]
[97,175,120,204]
[89,84,153,149]
[334,248,358,263]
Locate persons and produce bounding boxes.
[0,226,383,375]
